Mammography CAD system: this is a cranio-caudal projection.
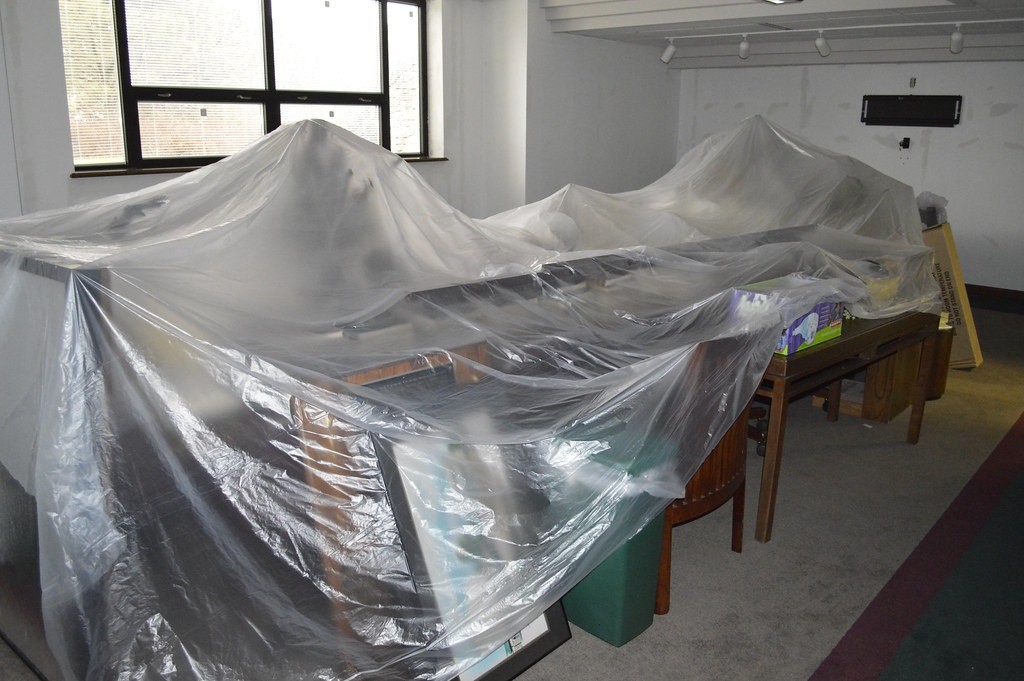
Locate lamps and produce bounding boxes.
[659,17,964,64]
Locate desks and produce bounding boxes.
[752,302,943,545]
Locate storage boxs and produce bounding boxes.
[921,221,984,369]
[729,271,843,356]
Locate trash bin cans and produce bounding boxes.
[545,424,675,649]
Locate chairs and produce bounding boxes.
[653,330,749,613]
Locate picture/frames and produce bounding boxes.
[369,373,573,680]
